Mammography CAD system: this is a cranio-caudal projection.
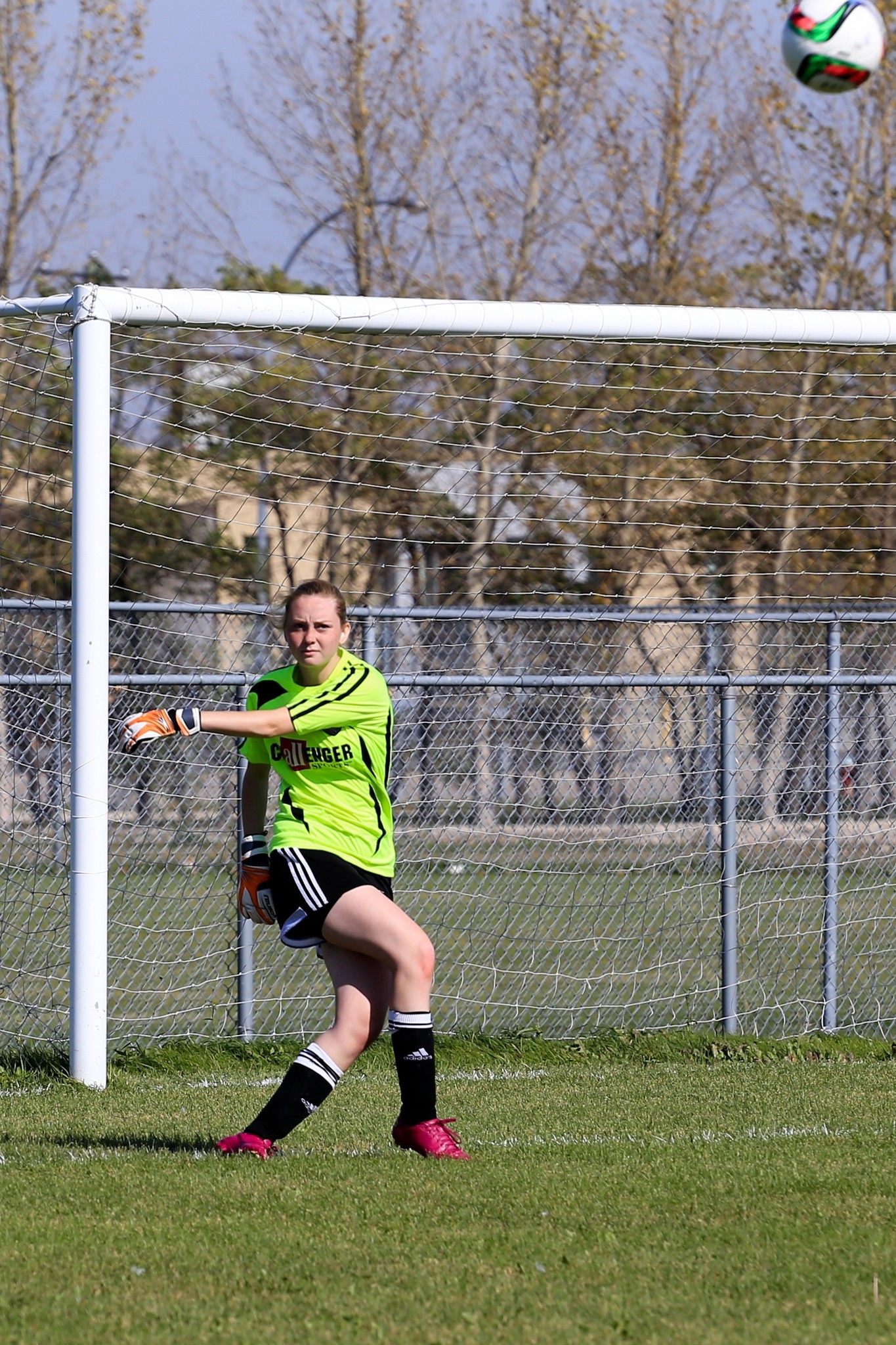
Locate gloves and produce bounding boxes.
[237,830,278,925]
[116,706,202,754]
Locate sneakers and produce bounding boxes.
[391,1115,472,1161]
[215,1133,273,1160]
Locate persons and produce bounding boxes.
[4,622,68,827]
[117,578,473,1164]
[125,639,197,826]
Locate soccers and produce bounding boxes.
[782,0,887,92]
